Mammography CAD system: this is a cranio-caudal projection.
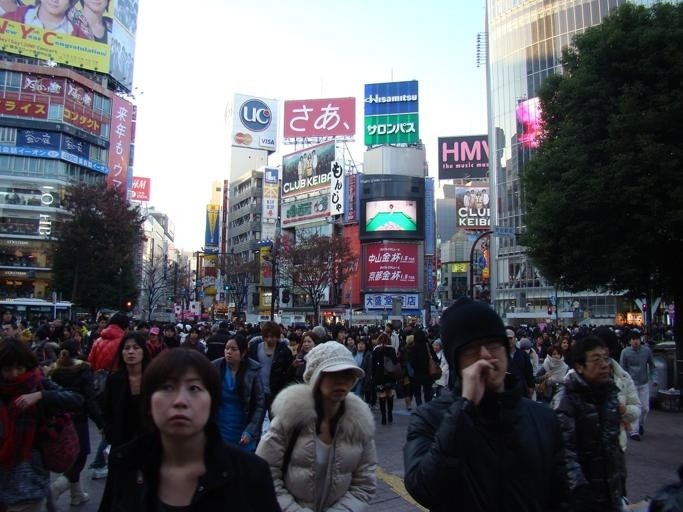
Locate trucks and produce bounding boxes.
[199,301,422,332]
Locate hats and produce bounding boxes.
[304,341,367,393]
[150,327,161,337]
[406,335,415,345]
[433,297,532,389]
[312,325,326,338]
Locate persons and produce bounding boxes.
[0,0,26,17]
[475,190,482,210]
[281,150,334,193]
[79,1,112,44]
[0,296,659,512]
[480,189,490,209]
[1,0,89,42]
[469,189,476,208]
[463,191,470,207]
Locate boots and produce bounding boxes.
[47,475,89,505]
[369,396,413,425]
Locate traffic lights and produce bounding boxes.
[126,301,131,310]
[547,306,551,314]
[223,284,236,290]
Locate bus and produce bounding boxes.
[0,298,76,328]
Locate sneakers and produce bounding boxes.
[87,464,109,481]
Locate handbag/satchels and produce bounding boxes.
[429,358,443,380]
[93,369,108,398]
[383,355,402,376]
[40,414,81,474]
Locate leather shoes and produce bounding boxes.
[630,425,644,442]
